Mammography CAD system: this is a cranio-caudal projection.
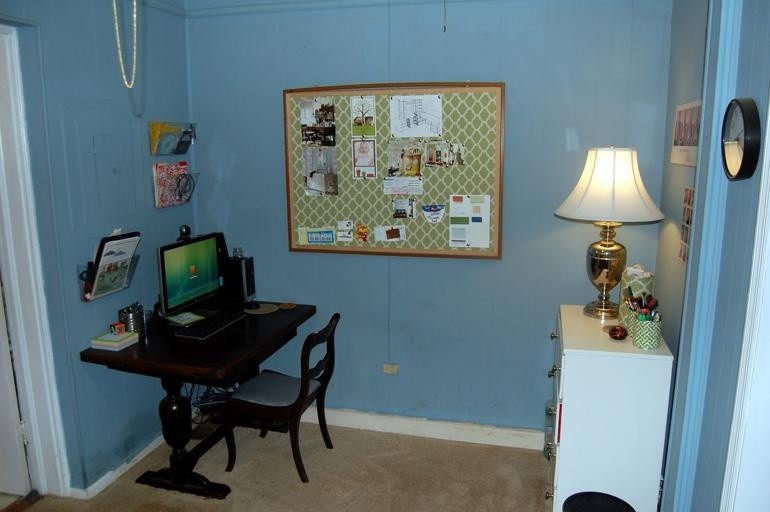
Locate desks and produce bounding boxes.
[81,299,317,499]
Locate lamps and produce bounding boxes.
[554,145,668,321]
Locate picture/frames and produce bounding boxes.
[281,81,503,263]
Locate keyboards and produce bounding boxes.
[174,310,247,341]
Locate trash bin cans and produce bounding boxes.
[562,492,636,512]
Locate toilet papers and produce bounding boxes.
[618,262,657,333]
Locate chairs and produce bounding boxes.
[217,312,342,483]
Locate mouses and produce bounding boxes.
[245,301,259,309]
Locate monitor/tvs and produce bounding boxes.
[156,231,221,316]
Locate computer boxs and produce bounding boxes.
[224,256,256,305]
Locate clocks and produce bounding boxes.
[721,98,761,181]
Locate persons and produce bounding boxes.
[354,142,375,167]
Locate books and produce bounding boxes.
[90,329,140,353]
[153,160,190,208]
[151,123,182,156]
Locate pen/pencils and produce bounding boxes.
[625,286,661,321]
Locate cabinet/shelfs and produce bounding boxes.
[543,304,675,512]
